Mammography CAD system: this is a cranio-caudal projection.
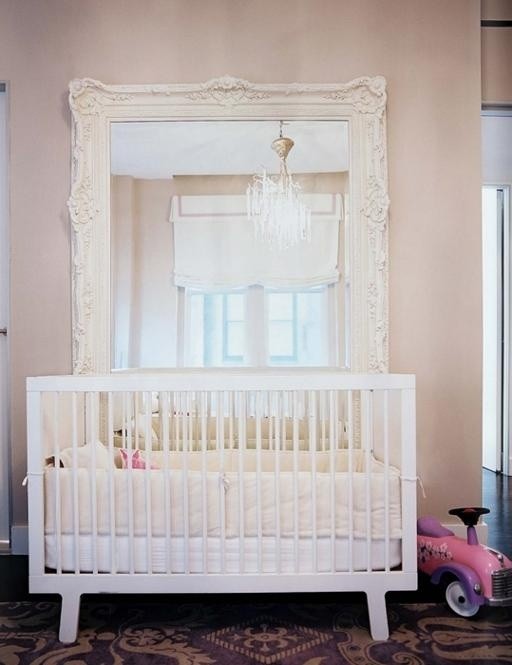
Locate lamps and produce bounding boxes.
[246,120,313,252]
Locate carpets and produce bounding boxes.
[0,603,511,664]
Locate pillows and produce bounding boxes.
[125,412,158,438]
[59,438,118,469]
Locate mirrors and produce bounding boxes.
[67,75,389,450]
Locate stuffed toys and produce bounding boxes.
[118,447,160,470]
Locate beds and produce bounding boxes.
[26,375,418,644]
